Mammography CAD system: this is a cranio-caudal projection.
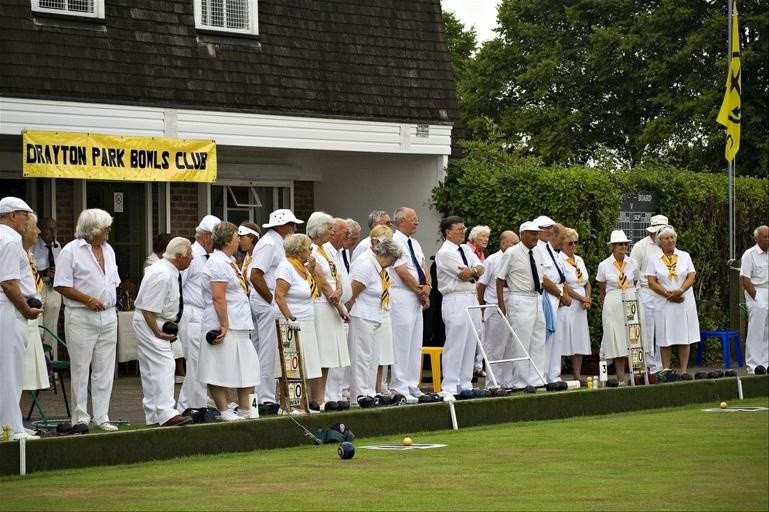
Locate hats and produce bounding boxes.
[195,209,306,238]
[607,230,632,244]
[519,216,555,232]
[0,196,34,213]
[646,214,669,233]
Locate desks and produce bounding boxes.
[115,312,143,374]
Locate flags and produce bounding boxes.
[715,4,741,161]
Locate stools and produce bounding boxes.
[420,346,445,393]
[696,331,743,370]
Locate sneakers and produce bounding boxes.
[99,423,118,431]
[277,408,320,415]
[14,428,40,440]
[405,395,419,404]
[221,409,249,421]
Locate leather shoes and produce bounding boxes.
[164,416,192,425]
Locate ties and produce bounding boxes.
[173,273,183,323]
[458,247,474,283]
[342,249,349,273]
[408,239,426,285]
[46,245,55,280]
[529,251,540,291]
[546,244,565,284]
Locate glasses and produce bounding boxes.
[657,225,673,234]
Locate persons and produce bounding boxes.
[247,206,432,415]
[596,215,701,386]
[53,210,121,432]
[132,214,260,425]
[0,197,62,438]
[740,225,769,375]
[434,217,590,400]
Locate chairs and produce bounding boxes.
[24,319,70,421]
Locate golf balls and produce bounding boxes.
[206,330,220,343]
[417,382,567,402]
[606,366,769,389]
[338,441,354,458]
[718,401,728,410]
[163,321,178,335]
[27,297,41,307]
[56,422,87,436]
[357,394,406,408]
[309,399,350,412]
[403,437,412,445]
